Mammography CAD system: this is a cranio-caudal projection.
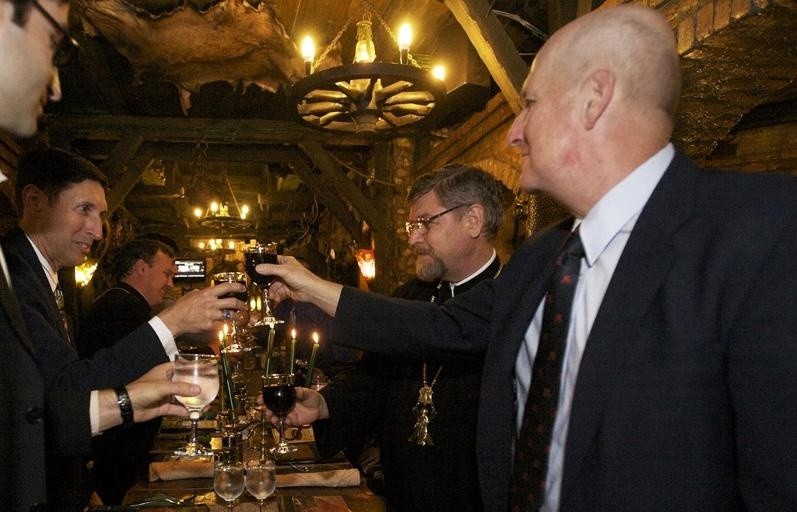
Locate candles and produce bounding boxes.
[217,323,235,411]
[264,321,320,386]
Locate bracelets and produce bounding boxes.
[114,382,134,425]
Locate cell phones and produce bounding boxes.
[274,441,347,464]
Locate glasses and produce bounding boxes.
[405,203,472,239]
[29,0,81,67]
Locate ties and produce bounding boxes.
[52,283,72,344]
[506,223,585,512]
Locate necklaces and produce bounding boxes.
[407,262,505,446]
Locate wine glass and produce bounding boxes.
[214,463,276,512]
[213,242,289,354]
[169,350,218,460]
[262,373,298,454]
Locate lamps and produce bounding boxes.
[286,2,450,140]
[191,154,256,258]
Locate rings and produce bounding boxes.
[223,311,231,321]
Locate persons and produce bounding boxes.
[1,0,211,512]
[255,0,797,512]
[135,231,249,344]
[256,160,517,511]
[80,236,242,506]
[15,145,249,390]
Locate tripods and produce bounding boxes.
[174,257,207,283]
